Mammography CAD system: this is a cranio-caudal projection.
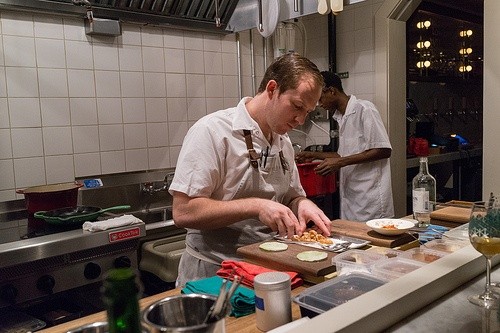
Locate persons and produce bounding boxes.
[168,51,332,288]
[293,71,395,218]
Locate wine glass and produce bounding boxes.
[467,200,500,310]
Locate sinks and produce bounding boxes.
[125,206,174,236]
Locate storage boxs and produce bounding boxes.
[296,162,336,197]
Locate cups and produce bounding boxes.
[142,294,231,333]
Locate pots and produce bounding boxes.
[33,204,131,227]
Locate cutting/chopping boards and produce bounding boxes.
[312,218,420,249]
[236,239,357,277]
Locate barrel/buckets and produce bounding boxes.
[15,181,84,238]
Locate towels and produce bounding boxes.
[179,275,256,318]
[215,259,304,292]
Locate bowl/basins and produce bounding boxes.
[365,218,415,235]
[293,222,469,319]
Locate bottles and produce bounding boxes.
[100,268,142,333]
[412,157,436,228]
[253,270,292,332]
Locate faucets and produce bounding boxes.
[144,186,154,195]
[162,177,169,191]
